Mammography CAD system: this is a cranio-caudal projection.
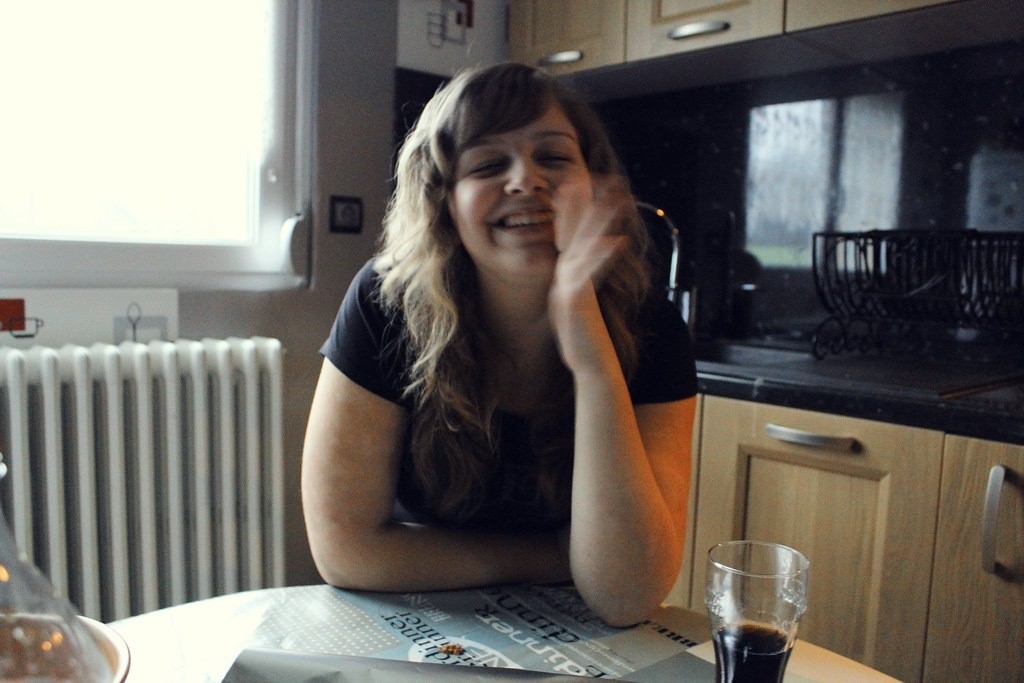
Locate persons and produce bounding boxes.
[301,62,698,628]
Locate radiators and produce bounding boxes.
[0,334,285,623]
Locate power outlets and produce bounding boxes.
[328,195,364,235]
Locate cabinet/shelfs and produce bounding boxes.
[660,393,1024,683]
[508,0,1024,101]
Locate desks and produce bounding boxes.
[102,584,905,683]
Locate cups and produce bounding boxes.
[0,612,132,683]
[704,539,811,683]
